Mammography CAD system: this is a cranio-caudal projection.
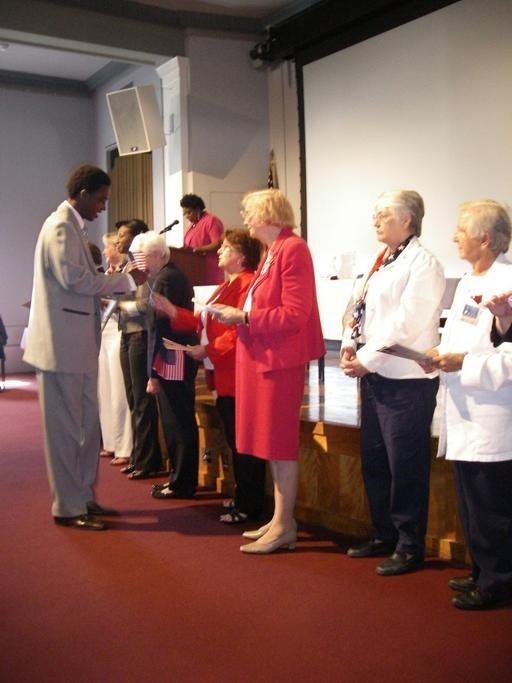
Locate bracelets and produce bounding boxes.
[241,310,246,324]
[245,311,249,324]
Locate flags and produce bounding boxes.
[265,168,274,189]
[127,248,148,273]
[149,339,186,382]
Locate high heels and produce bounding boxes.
[240,528,298,554]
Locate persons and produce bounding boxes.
[19,163,150,530]
[150,229,270,524]
[338,189,447,577]
[0,318,10,392]
[207,189,326,553]
[85,216,201,497]
[419,202,512,613]
[177,194,224,284]
[485,285,512,346]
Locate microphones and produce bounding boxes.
[160,219,180,234]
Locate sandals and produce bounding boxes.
[216,499,243,511]
[219,509,269,523]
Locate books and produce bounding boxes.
[378,340,431,363]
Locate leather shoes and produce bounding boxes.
[242,518,298,539]
[376,549,426,576]
[347,537,395,558]
[86,502,119,516]
[449,575,480,591]
[100,449,196,498]
[451,592,511,610]
[54,514,106,531]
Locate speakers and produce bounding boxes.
[104,84,168,157]
